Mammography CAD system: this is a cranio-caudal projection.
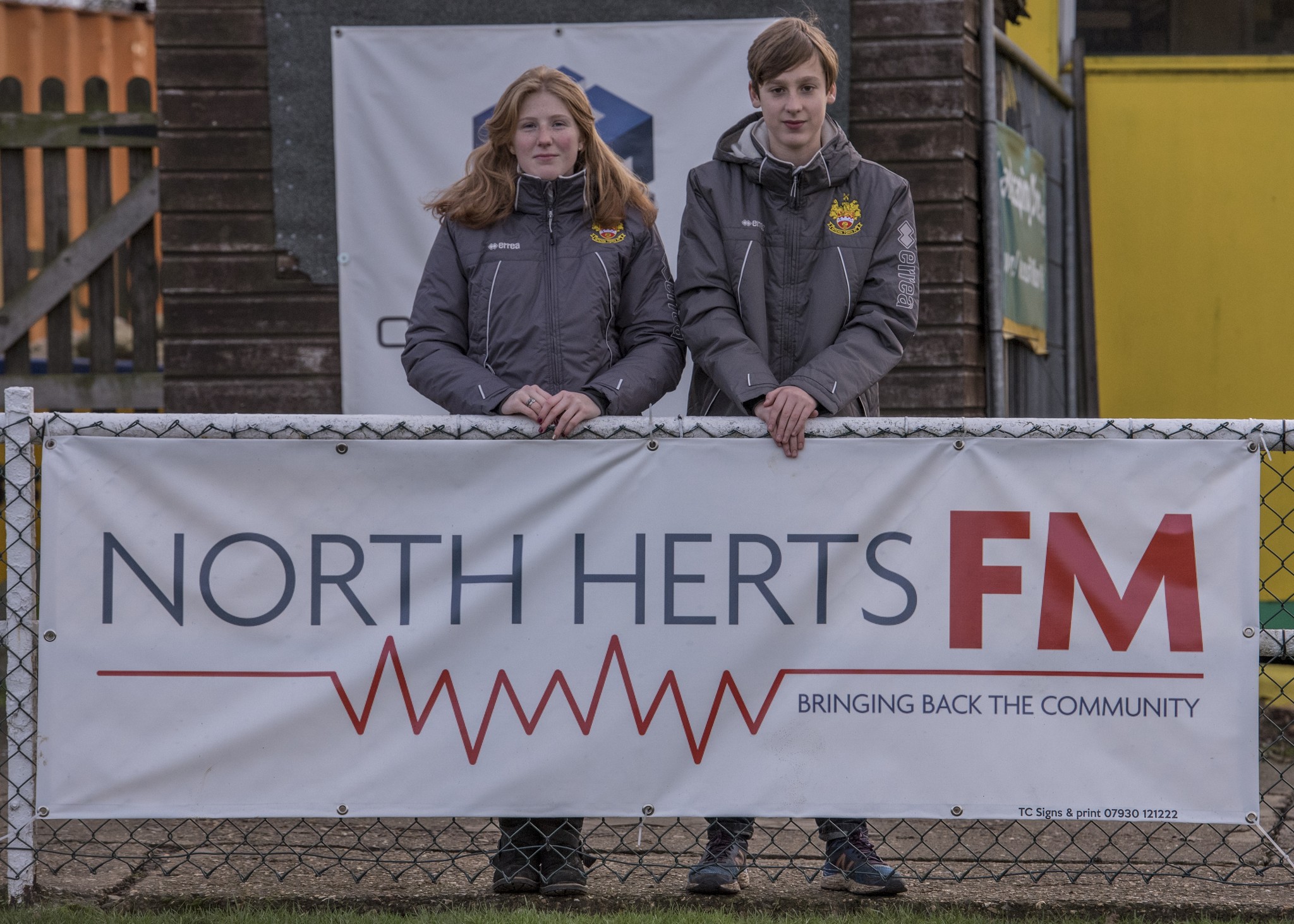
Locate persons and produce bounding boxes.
[403,66,687,896]
[674,16,921,897]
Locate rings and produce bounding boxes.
[526,398,533,406]
[528,399,535,408]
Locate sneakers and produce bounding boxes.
[493,856,538,894]
[686,837,751,892]
[539,846,588,892]
[819,827,908,896]
